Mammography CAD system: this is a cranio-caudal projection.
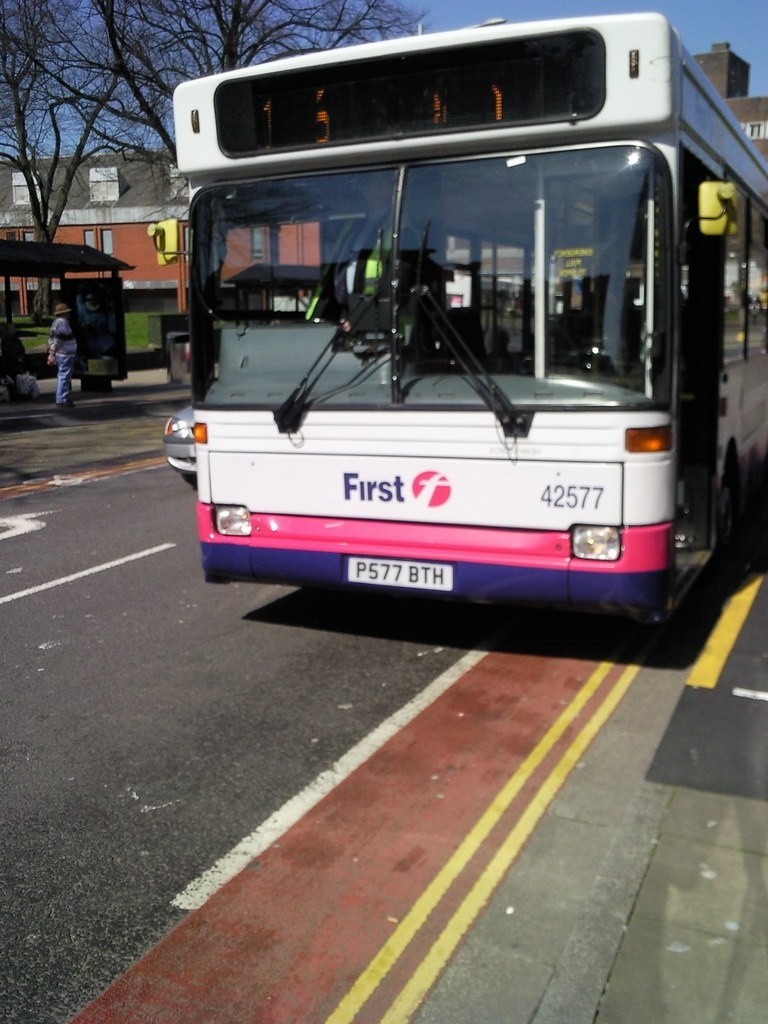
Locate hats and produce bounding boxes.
[54,303,72,316]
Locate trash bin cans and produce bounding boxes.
[165,330,192,390]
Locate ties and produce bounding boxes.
[335,215,366,303]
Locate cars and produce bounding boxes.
[162,404,197,478]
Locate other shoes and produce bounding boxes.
[15,396,25,401]
[56,400,75,409]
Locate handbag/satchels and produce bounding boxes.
[0,374,14,385]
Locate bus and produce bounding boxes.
[146,11,768,629]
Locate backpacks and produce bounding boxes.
[16,370,40,400]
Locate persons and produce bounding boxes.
[336,175,422,350]
[1,323,26,401]
[48,304,77,408]
